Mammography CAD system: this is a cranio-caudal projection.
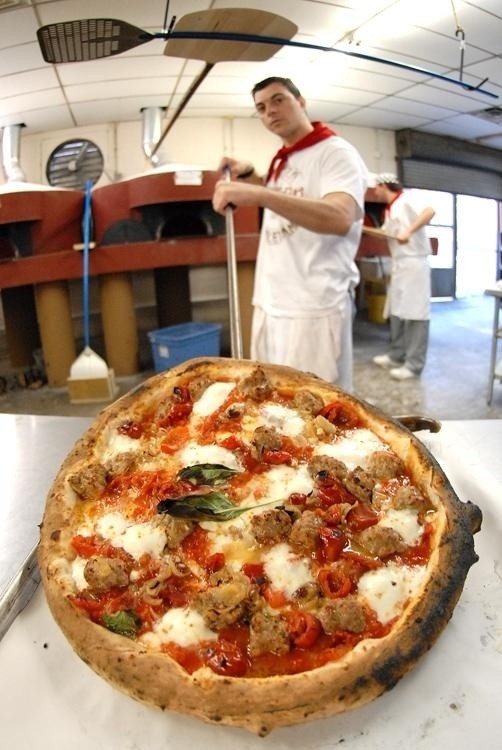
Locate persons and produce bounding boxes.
[212,77,370,395]
[371,173,436,381]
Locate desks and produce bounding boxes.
[481,279,502,406]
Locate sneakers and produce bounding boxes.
[373,354,415,379]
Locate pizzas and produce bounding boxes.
[39,355,482,738]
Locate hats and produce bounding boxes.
[375,173,400,184]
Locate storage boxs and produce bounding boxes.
[67,368,120,405]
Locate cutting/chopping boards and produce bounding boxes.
[144,7,299,158]
